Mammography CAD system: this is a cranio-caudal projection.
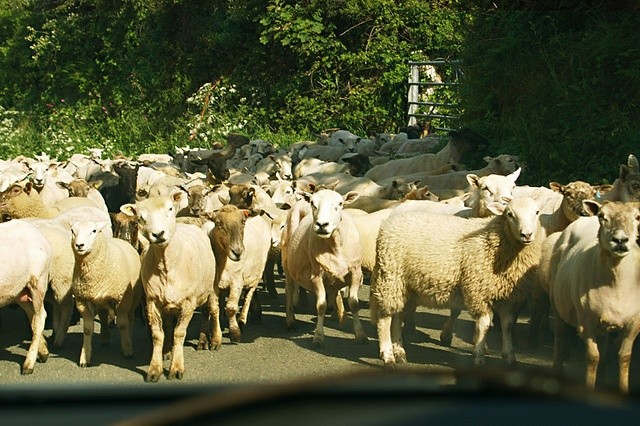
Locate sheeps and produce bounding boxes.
[56,206,113,235]
[375,179,422,201]
[269,171,294,207]
[16,220,54,376]
[294,173,380,196]
[293,153,373,179]
[302,137,361,162]
[375,132,393,151]
[267,152,293,181]
[64,147,105,177]
[88,159,139,214]
[266,215,287,298]
[68,219,143,368]
[54,179,104,199]
[329,131,377,153]
[376,154,522,202]
[601,153,640,202]
[222,184,279,216]
[395,136,441,157]
[173,184,221,218]
[137,145,190,185]
[41,160,69,176]
[541,180,615,237]
[553,199,639,399]
[363,131,469,185]
[236,138,267,184]
[190,152,236,185]
[0,173,75,349]
[342,185,441,214]
[33,151,50,162]
[285,191,371,348]
[368,194,546,371]
[119,190,223,383]
[21,161,57,199]
[389,166,522,347]
[187,131,250,161]
[197,204,271,342]
